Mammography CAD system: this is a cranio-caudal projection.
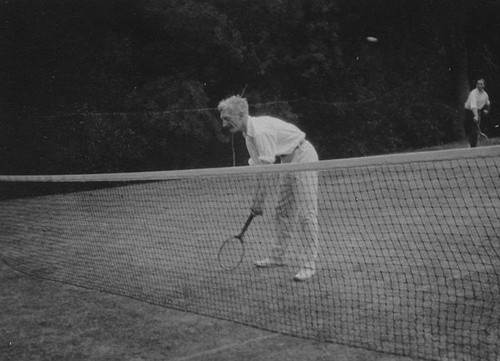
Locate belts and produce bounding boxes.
[280,141,303,156]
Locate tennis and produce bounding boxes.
[367,36,378,41]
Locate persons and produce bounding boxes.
[217,94,320,282]
[463,80,491,148]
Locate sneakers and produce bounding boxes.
[293,268,315,281]
[254,255,288,266]
